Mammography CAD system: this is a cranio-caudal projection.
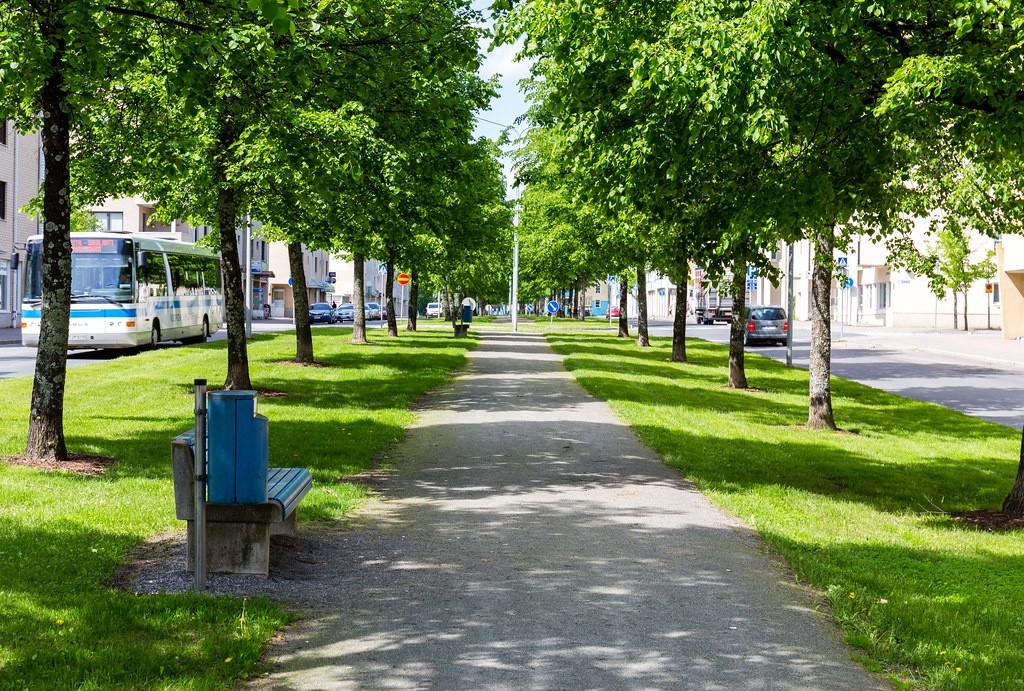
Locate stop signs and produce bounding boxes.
[397,273,410,286]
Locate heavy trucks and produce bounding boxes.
[695,283,751,325]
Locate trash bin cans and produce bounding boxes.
[462,306,472,322]
[207,390,269,506]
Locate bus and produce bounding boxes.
[21,232,223,351]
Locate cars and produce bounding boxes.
[309,303,387,324]
[744,306,788,346]
[426,303,442,318]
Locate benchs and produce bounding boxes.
[171,421,311,579]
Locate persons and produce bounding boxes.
[332,301,336,308]
